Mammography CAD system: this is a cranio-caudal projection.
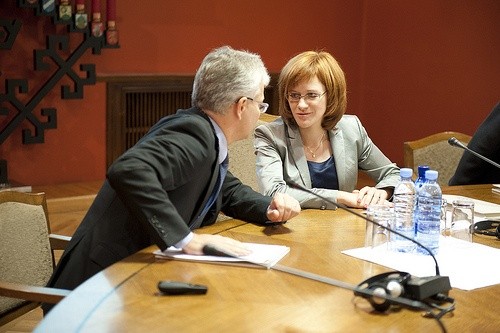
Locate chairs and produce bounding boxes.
[0,190,72,325]
[216,113,281,221]
[403,131,472,184]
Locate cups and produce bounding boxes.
[365,205,389,247]
[450,201,474,242]
[439,198,447,237]
[372,211,392,247]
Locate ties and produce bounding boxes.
[189,154,230,231]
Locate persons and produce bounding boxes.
[40,45,302,318]
[254,50,401,210]
[448,103,500,186]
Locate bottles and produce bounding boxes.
[413,166,429,235]
[393,167,415,253]
[416,169,442,256]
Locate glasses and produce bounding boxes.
[235,96,269,116]
[284,90,327,102]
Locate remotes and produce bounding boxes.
[158,281,207,296]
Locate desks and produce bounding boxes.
[34,184,500,333]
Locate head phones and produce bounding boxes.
[353,272,410,312]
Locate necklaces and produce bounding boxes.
[303,131,327,157]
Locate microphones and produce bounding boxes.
[448,136,500,168]
[286,179,452,306]
